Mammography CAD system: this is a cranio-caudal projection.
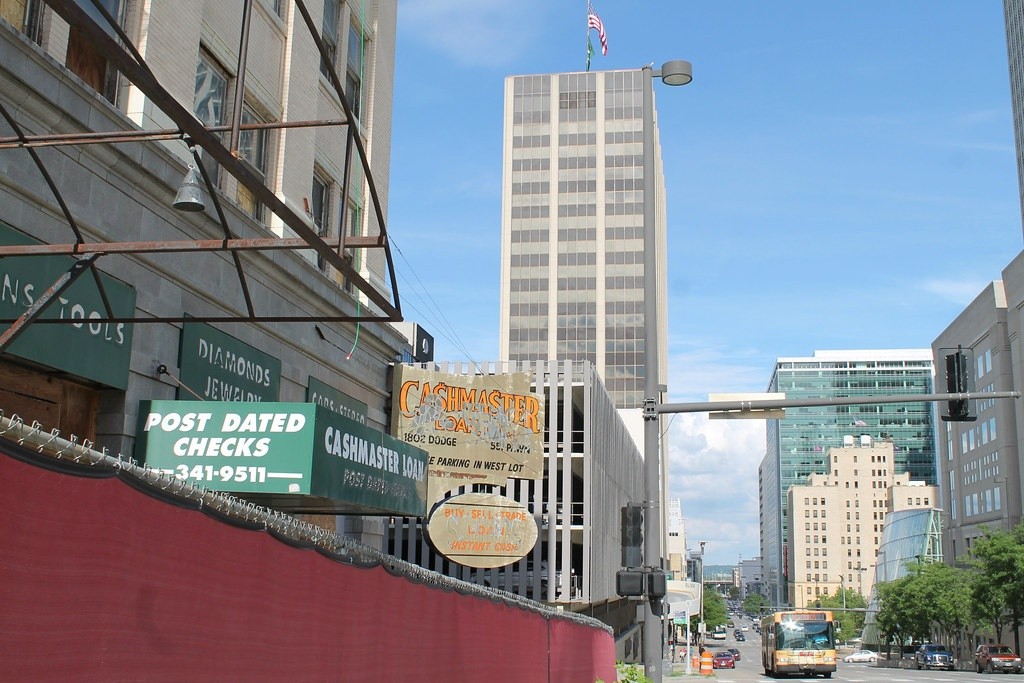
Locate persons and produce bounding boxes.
[667,641,705,670]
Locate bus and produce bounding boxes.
[760,611,841,678]
[711,623,726,639]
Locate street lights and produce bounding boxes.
[642,55,694,682]
[993,477,1010,537]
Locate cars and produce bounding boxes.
[727,622,735,627]
[842,649,878,663]
[752,623,762,635]
[875,551,886,585]
[711,651,736,669]
[728,648,740,661]
[746,612,760,620]
[733,629,741,636]
[734,631,744,638]
[974,644,1021,675]
[726,606,743,619]
[736,634,746,641]
[741,624,748,632]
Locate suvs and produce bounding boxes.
[915,644,954,670]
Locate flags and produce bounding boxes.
[588,0,608,56]
[588,34,595,56]
[814,445,822,452]
